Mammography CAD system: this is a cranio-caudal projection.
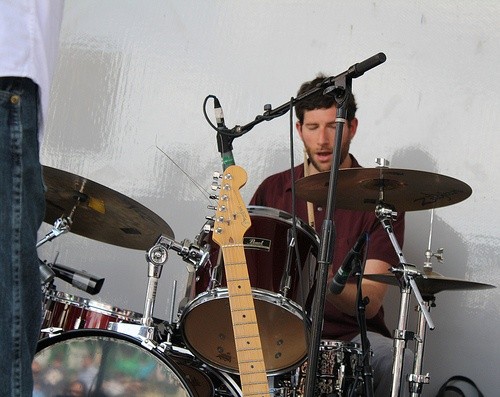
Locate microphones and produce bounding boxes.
[330,231,368,295]
[213,98,236,171]
[38,260,55,282]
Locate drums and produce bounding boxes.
[273,339,374,397]
[38,289,180,348]
[30,328,244,397]
[181,204,323,376]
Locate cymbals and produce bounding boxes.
[294,168,473,212]
[41,164,175,251]
[363,272,497,298]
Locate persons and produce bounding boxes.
[196,72,418,397]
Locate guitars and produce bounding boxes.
[204,165,272,396]
